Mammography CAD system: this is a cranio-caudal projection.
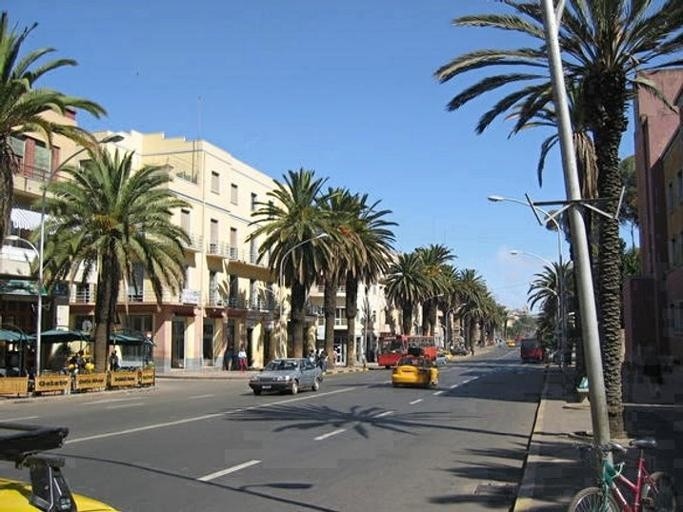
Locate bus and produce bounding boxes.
[377,332,437,369]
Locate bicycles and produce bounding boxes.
[567,435,677,512]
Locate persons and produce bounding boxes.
[110,351,121,369]
[225,349,234,371]
[309,346,338,372]
[238,346,249,371]
[69,350,87,374]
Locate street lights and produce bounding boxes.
[258,232,329,356]
[488,195,565,373]
[416,294,480,350]
[364,272,404,354]
[4,134,124,377]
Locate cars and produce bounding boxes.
[391,348,469,388]
[249,357,323,395]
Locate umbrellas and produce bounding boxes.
[0,329,35,345]
[108,333,143,345]
[28,328,91,342]
